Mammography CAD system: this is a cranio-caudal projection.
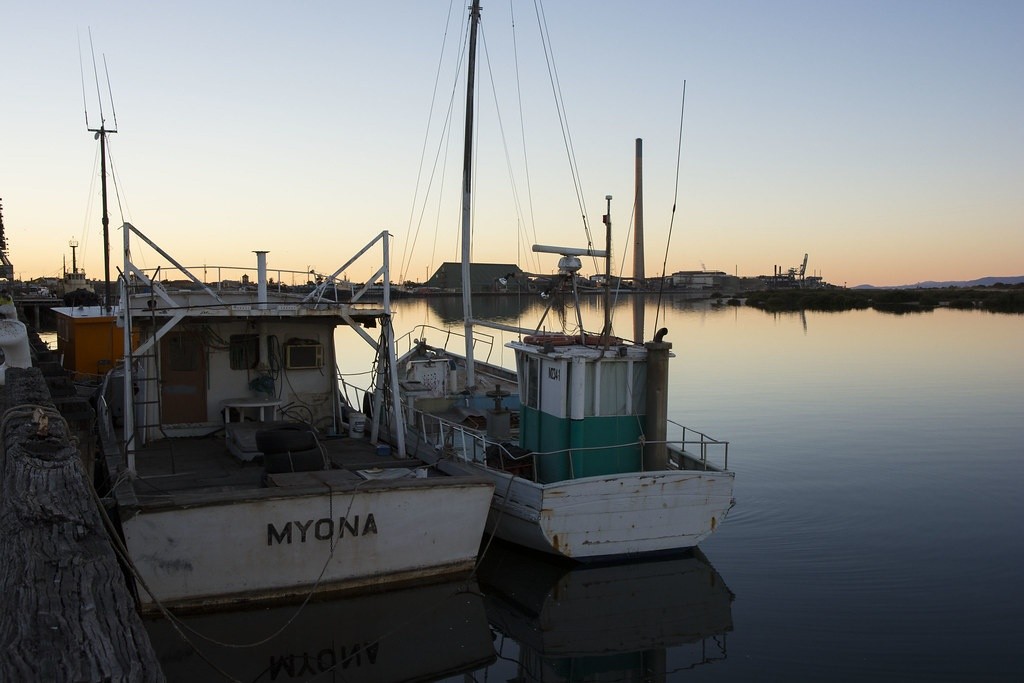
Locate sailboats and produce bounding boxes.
[42,1,736,618]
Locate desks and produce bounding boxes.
[399,381,432,426]
[224,399,281,422]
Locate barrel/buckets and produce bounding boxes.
[349,413,367,439]
